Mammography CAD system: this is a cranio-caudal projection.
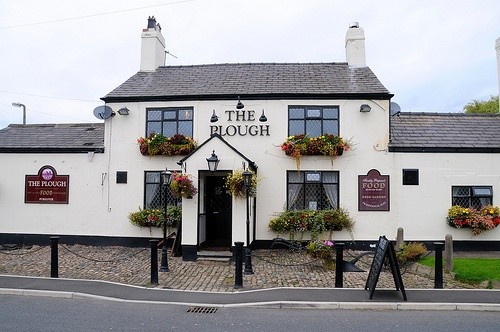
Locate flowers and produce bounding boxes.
[446,203,500,235]
[170,171,198,198]
[225,167,260,200]
[270,208,355,270]
[137,132,198,159]
[131,204,182,232]
[281,133,351,176]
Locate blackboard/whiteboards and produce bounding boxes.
[366,237,404,294]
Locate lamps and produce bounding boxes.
[211,110,218,122]
[118,108,129,115]
[259,109,267,122]
[237,96,244,109]
[206,150,220,171]
[360,105,371,112]
